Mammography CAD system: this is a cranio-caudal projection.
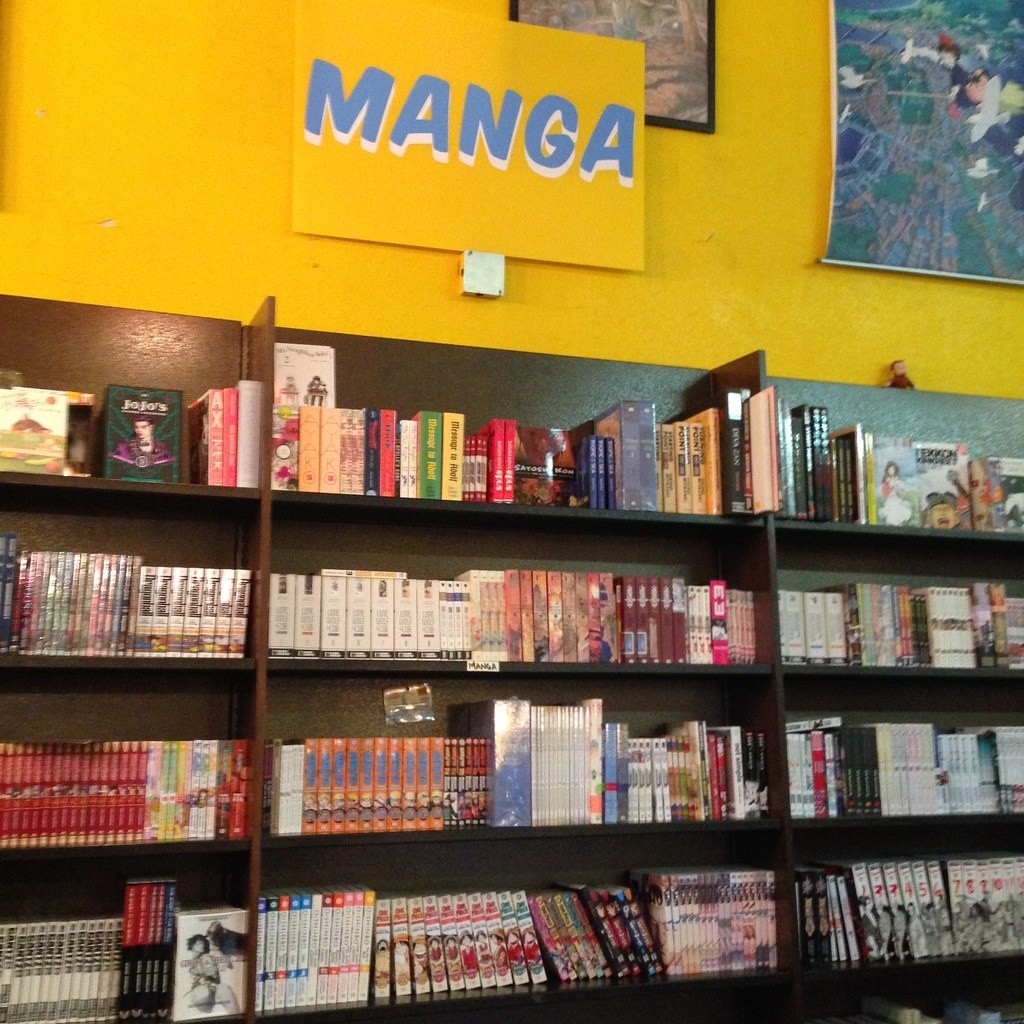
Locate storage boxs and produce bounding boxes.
[445,700,531,826]
[170,908,249,1021]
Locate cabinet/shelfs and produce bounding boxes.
[0,294,1024,1024]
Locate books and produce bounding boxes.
[776,581,1024,669]
[784,404,1024,532]
[273,344,786,518]
[0,377,259,1024]
[786,710,1024,819]
[266,570,756,665]
[256,866,775,1013]
[263,698,771,836]
[793,851,1024,963]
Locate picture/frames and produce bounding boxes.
[509,0,716,134]
